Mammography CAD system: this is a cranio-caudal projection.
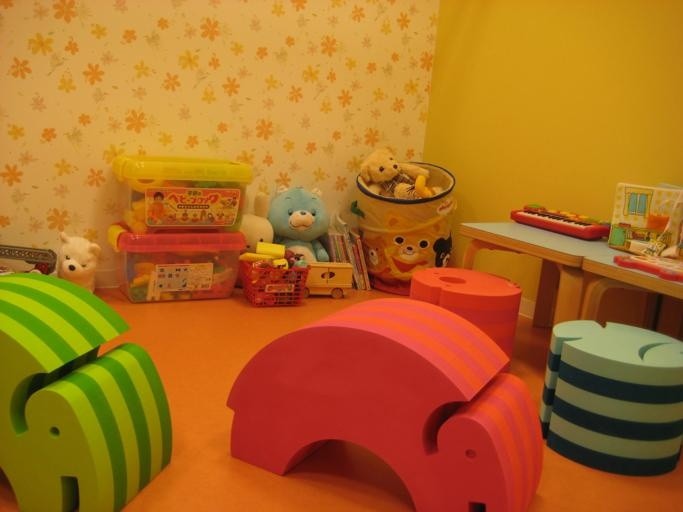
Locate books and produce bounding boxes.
[327,214,372,291]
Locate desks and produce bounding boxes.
[456,218,613,327]
[581,254,683,322]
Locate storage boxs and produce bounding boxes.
[109,221,245,305]
[113,156,252,234]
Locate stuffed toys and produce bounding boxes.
[56,230,100,294]
[240,213,275,252]
[358,144,440,199]
[267,186,329,264]
